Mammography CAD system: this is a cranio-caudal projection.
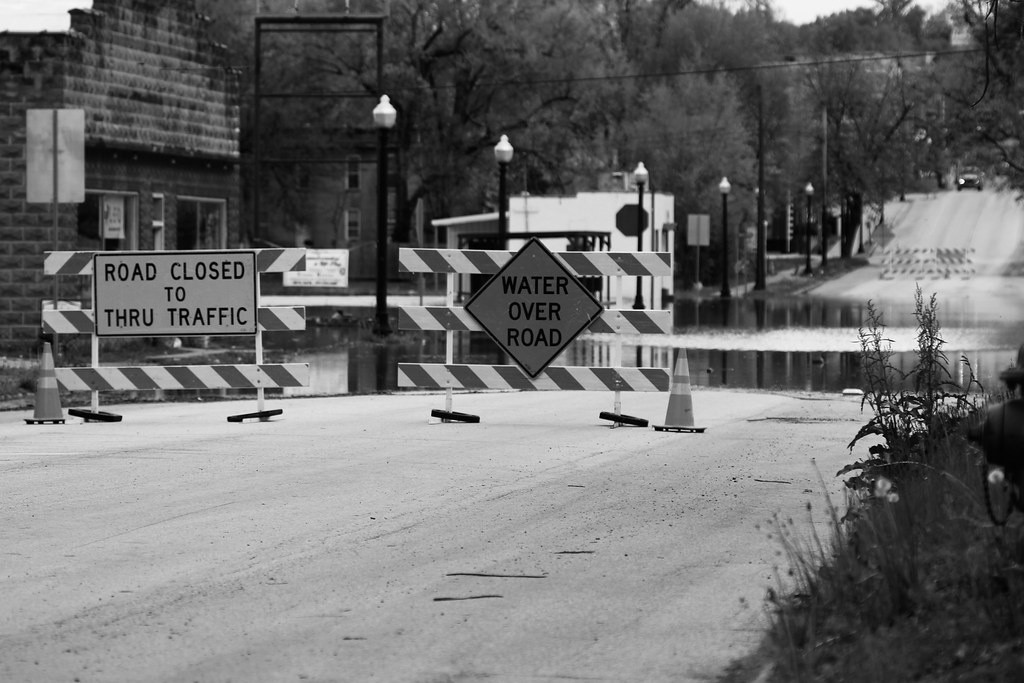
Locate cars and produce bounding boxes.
[957,168,983,191]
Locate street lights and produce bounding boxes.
[372,95,396,337]
[495,134,514,250]
[633,162,647,310]
[804,182,814,273]
[718,176,731,298]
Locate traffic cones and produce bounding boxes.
[23,342,66,424]
